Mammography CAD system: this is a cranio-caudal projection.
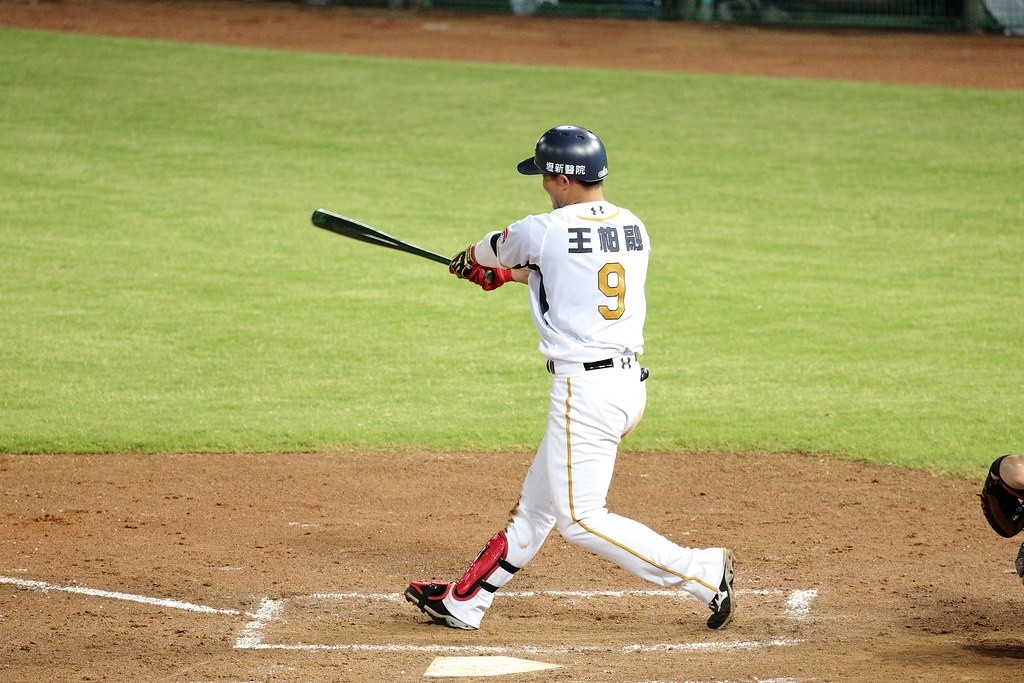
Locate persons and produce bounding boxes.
[980,454,1024,580]
[404,126,738,631]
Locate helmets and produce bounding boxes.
[517,125,608,183]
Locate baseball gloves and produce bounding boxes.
[975,453,1024,539]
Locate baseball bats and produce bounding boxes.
[310,206,496,285]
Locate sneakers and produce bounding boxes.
[706,547,736,630]
[404,583,476,630]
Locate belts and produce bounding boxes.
[547,358,613,374]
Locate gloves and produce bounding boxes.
[470,266,516,291]
[449,245,478,279]
